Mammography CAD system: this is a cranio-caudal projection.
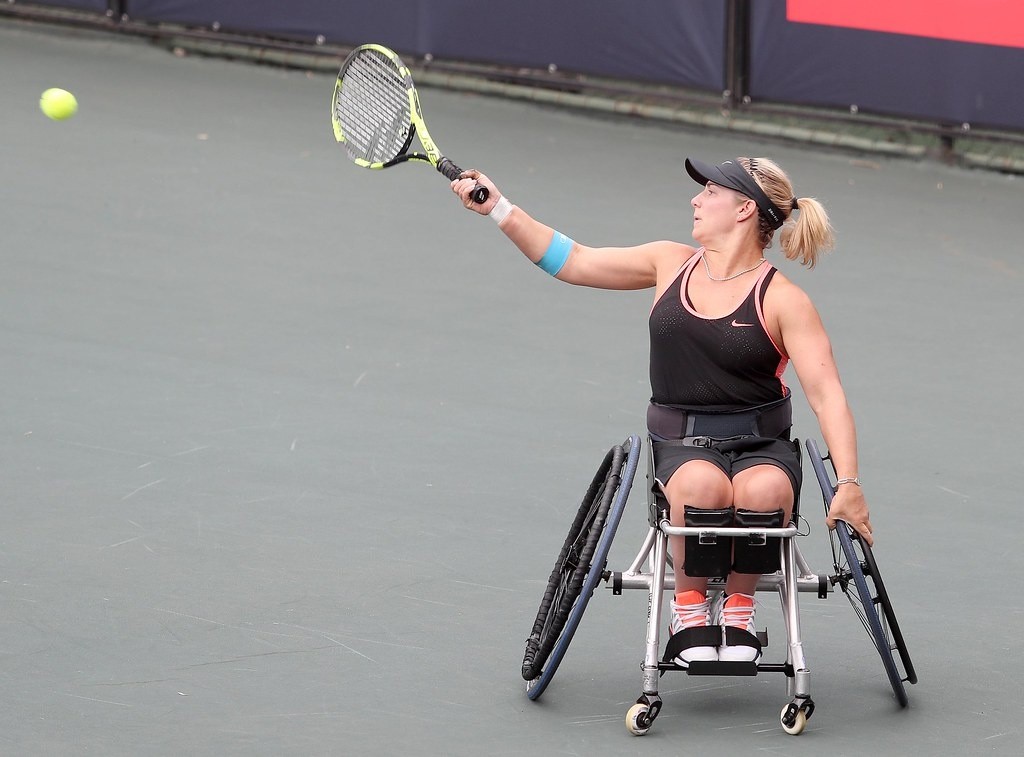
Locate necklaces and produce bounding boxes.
[700,253,767,281]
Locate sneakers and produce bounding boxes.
[710,588,766,668]
[667,590,719,668]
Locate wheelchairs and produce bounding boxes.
[520,435,918,736]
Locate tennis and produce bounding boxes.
[38,86,79,122]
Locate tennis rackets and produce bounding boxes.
[324,41,493,208]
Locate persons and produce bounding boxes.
[450,158,872,669]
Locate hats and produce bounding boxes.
[684,156,786,230]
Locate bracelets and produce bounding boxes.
[488,195,514,225]
[836,476,864,486]
